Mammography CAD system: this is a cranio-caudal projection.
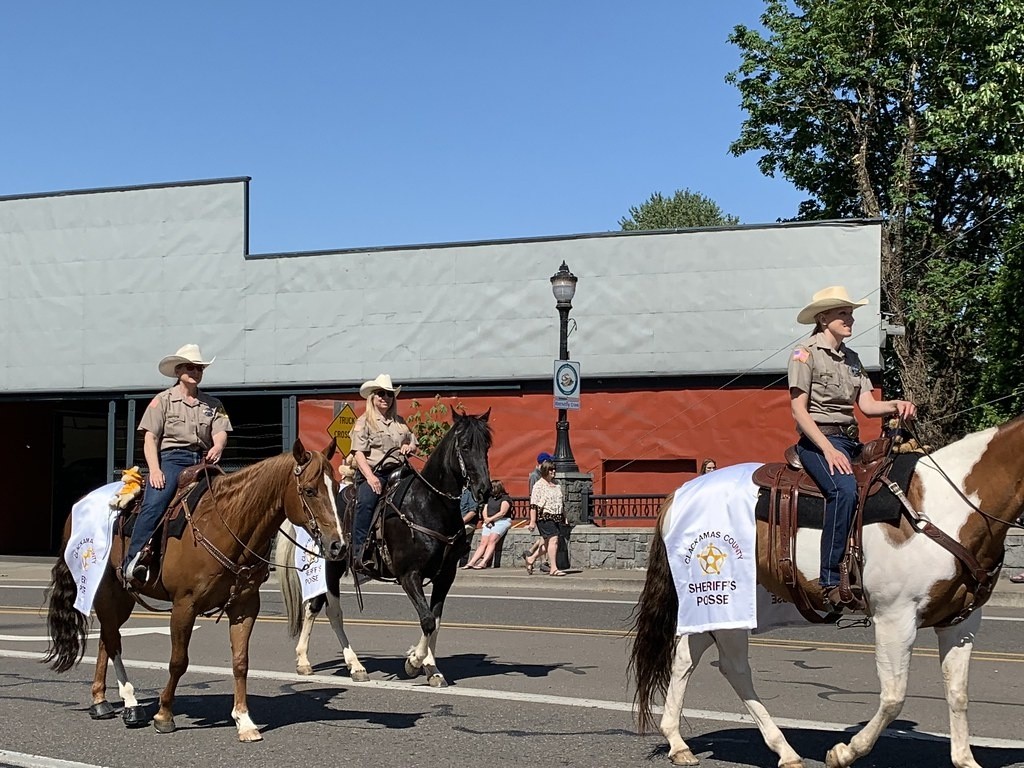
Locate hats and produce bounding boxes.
[359,373,403,399]
[795,285,870,324]
[536,452,556,463]
[159,343,215,377]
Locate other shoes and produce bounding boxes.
[828,584,863,603]
[119,565,146,585]
[539,562,551,573]
[522,550,532,560]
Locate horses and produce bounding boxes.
[273,404,495,687]
[619,416,1024,768]
[38,437,350,742]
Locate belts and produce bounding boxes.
[816,424,860,438]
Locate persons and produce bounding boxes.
[525,461,568,577]
[524,452,560,572]
[461,484,479,546]
[122,343,233,592]
[349,373,420,566]
[462,479,512,569]
[699,458,717,476]
[786,285,919,616]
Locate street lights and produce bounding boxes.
[550,260,580,473]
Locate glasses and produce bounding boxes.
[705,467,716,470]
[184,363,204,372]
[548,467,557,472]
[375,390,395,399]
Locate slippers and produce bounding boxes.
[523,555,533,575]
[473,563,487,569]
[549,570,567,577]
[460,563,472,570]
